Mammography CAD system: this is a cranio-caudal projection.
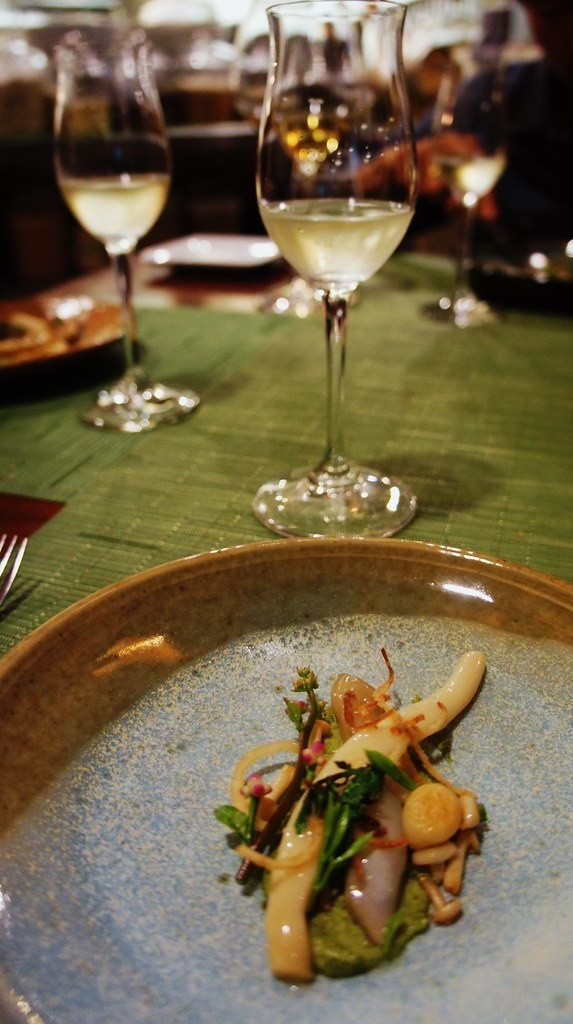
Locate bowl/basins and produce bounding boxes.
[462,255,573,312]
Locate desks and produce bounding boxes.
[0,232,573,670]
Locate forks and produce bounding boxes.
[0,534,28,607]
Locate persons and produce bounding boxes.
[359,0,573,316]
[299,38,462,249]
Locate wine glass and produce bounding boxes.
[53,25,201,435]
[249,1,419,538]
[418,47,508,329]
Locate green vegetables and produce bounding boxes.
[212,665,488,957]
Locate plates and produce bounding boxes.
[0,292,137,386]
[0,537,572,1024]
